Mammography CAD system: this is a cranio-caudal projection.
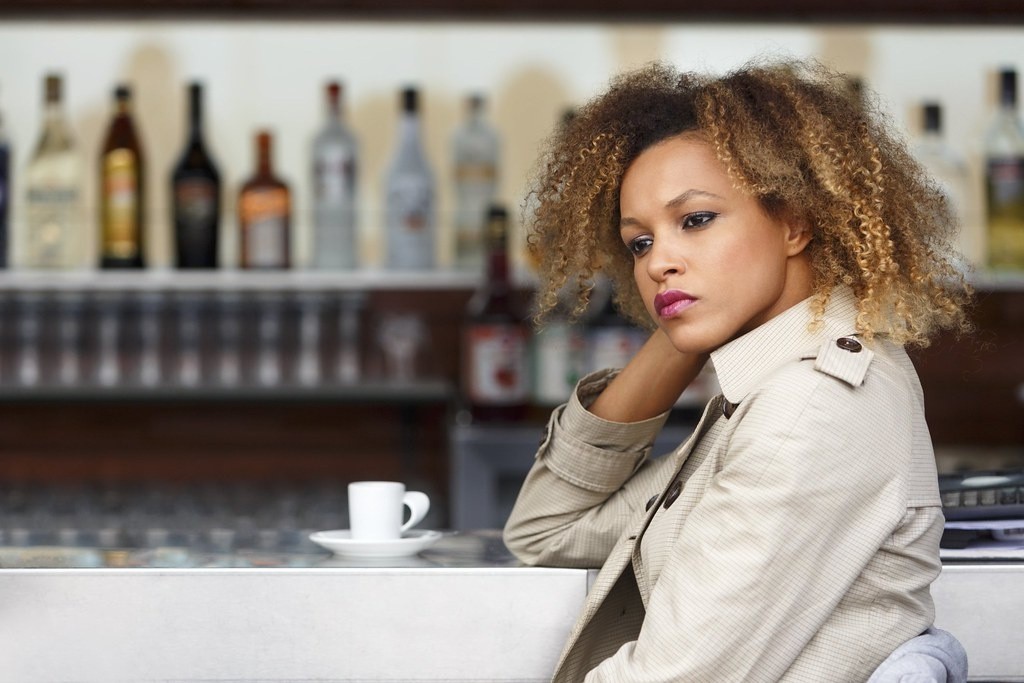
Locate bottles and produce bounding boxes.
[0,115,13,270]
[912,103,971,258]
[100,85,143,270]
[308,80,357,271]
[239,131,290,269]
[0,294,366,386]
[462,204,533,423]
[26,73,85,271]
[985,67,1024,273]
[533,290,586,426]
[452,95,502,272]
[171,84,222,267]
[586,286,650,370]
[381,86,436,270]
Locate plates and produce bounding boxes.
[309,530,443,557]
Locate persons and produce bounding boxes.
[500,53,963,682]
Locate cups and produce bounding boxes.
[348,482,430,540]
[380,319,424,386]
